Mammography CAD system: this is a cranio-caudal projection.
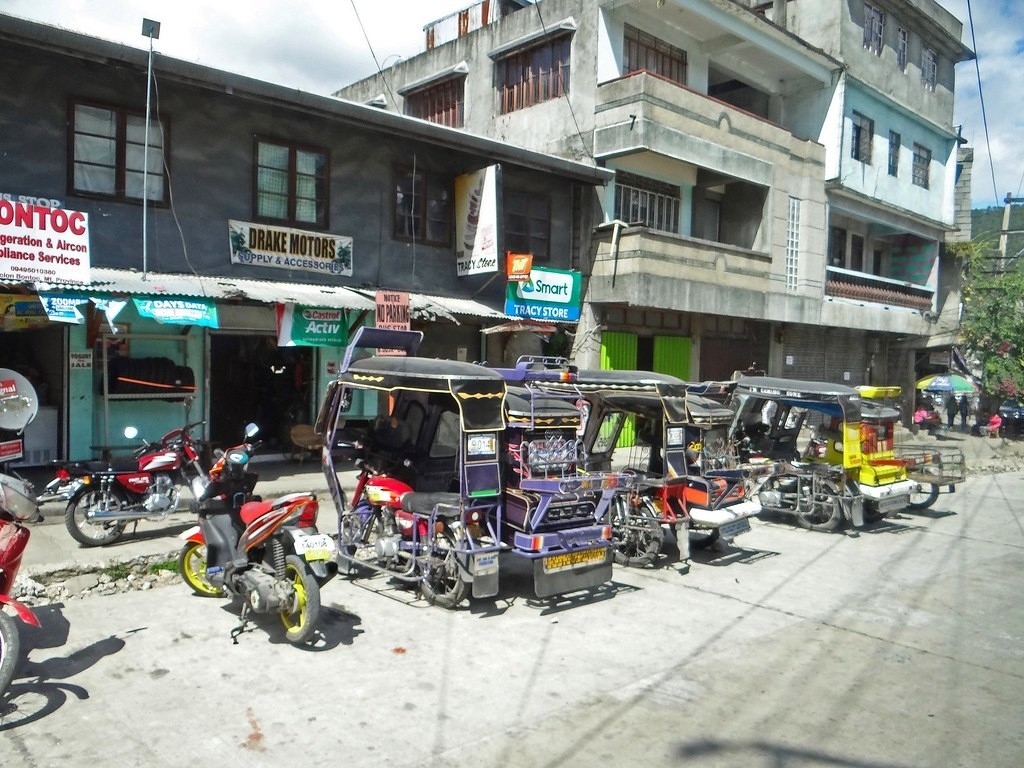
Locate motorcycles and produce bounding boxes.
[50,394,213,546]
[0,456,68,701]
[179,422,336,644]
[731,371,941,532]
[575,368,778,570]
[312,327,638,609]
[793,385,966,510]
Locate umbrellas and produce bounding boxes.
[912,373,980,391]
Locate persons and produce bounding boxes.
[915,396,969,435]
[979,412,1002,438]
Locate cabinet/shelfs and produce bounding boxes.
[89,333,199,469]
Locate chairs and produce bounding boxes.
[988,427,999,439]
[291,424,323,468]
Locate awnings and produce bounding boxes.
[2,264,580,326]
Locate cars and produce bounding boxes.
[1000,400,1024,423]
[935,394,962,407]
